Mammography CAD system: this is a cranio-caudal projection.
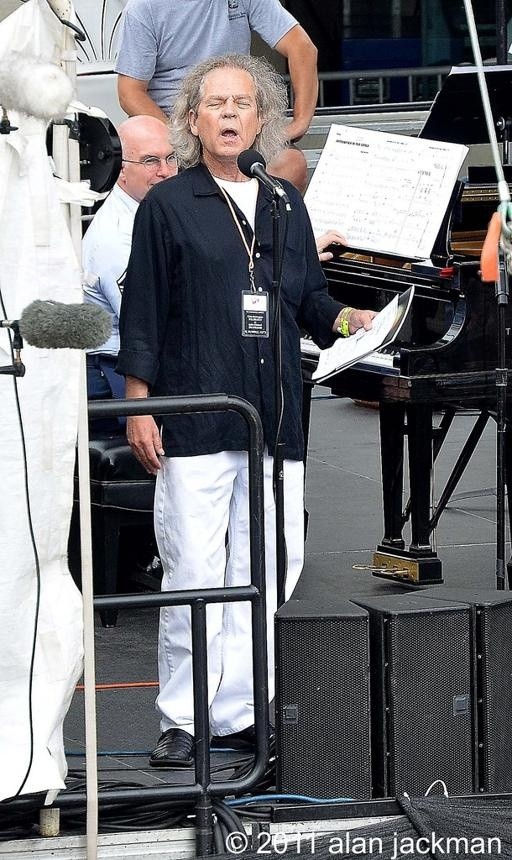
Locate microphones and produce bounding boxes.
[0,50,74,124]
[237,149,290,207]
[19,300,112,350]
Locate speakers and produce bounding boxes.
[406,584,512,798]
[274,595,371,805]
[349,593,481,800]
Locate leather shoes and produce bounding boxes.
[210,721,276,757]
[150,728,195,767]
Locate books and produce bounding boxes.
[309,282,415,384]
[301,122,472,261]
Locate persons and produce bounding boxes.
[79,113,348,428]
[111,53,382,772]
[115,1,323,195]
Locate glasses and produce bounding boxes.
[122,154,182,172]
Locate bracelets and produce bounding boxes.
[336,306,355,338]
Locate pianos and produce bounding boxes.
[297,183,512,591]
